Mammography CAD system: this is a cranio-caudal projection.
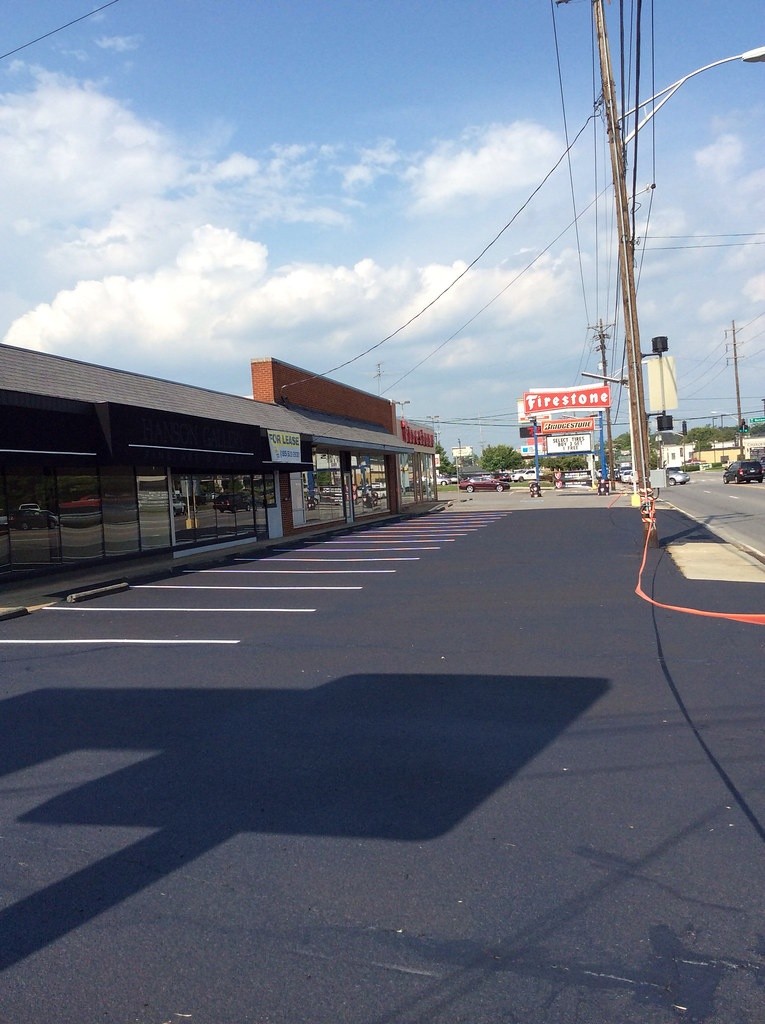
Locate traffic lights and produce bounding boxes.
[744,425,748,434]
[739,424,743,433]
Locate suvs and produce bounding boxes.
[722,459,764,485]
[212,492,253,513]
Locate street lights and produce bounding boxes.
[711,411,744,460]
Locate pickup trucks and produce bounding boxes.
[511,469,551,482]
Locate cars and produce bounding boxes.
[436,475,452,486]
[458,477,511,492]
[0,503,60,530]
[597,462,639,485]
[173,499,186,517]
[668,470,691,486]
[303,485,386,510]
[756,457,765,476]
[496,473,511,482]
[450,475,459,484]
